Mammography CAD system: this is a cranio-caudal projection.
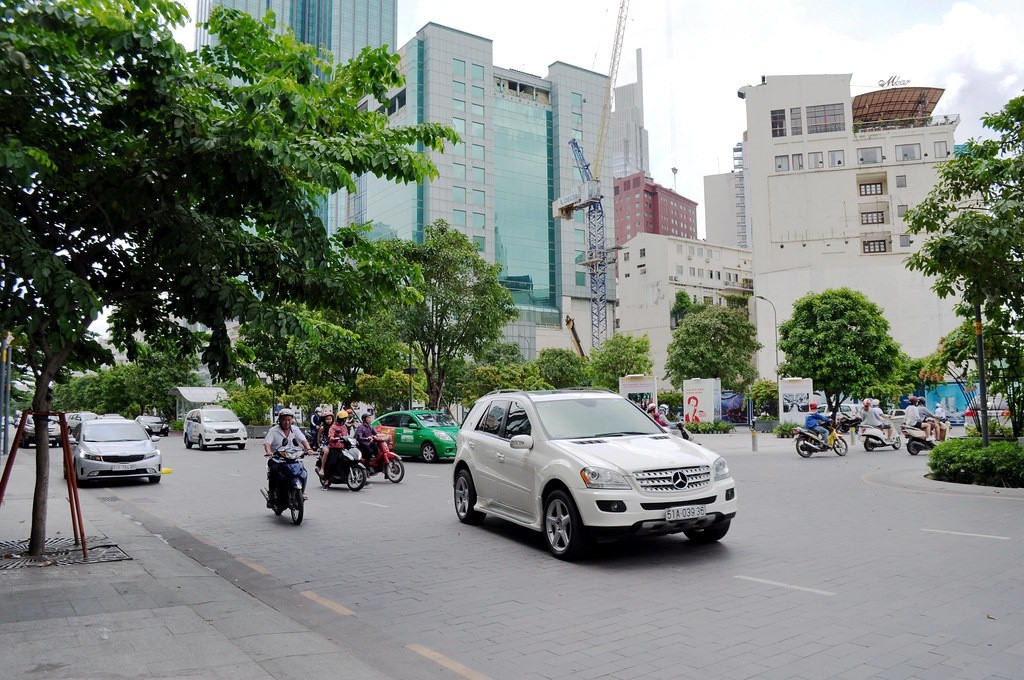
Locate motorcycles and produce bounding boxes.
[835,412,863,433]
[260,427,367,525]
[352,433,405,483]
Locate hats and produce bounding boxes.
[935,403,941,408]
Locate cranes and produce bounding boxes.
[551,0,630,349]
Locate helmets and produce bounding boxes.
[346,409,353,415]
[278,408,294,419]
[909,396,917,403]
[648,403,656,410]
[322,412,334,422]
[336,411,348,419]
[361,412,372,421]
[871,399,880,406]
[659,404,668,415]
[864,398,872,407]
[809,403,817,409]
[917,397,925,405]
[315,407,322,413]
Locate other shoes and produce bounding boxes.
[322,483,328,490]
[366,466,371,474]
[823,443,828,447]
[303,495,308,500]
[887,439,894,442]
[319,468,324,476]
[926,437,936,441]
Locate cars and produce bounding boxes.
[946,414,965,426]
[65,413,125,434]
[371,410,461,463]
[182,409,248,451]
[63,419,162,484]
[135,415,169,436]
[885,409,907,420]
[9,416,21,428]
[965,394,1018,430]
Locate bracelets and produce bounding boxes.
[307,449,313,450]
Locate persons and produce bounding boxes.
[353,412,378,479]
[861,398,894,441]
[916,397,941,441]
[903,396,930,437]
[685,396,700,423]
[262,408,314,508]
[935,403,943,416]
[311,407,323,446]
[805,402,833,449]
[317,411,349,491]
[654,404,679,429]
[647,404,656,419]
[345,409,355,435]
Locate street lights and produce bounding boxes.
[756,295,779,385]
[404,367,418,411]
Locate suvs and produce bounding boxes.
[452,387,737,561]
[817,403,864,421]
[17,413,61,448]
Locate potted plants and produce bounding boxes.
[853,115,951,134]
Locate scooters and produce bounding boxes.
[792,418,902,458]
[673,422,701,445]
[901,417,952,455]
